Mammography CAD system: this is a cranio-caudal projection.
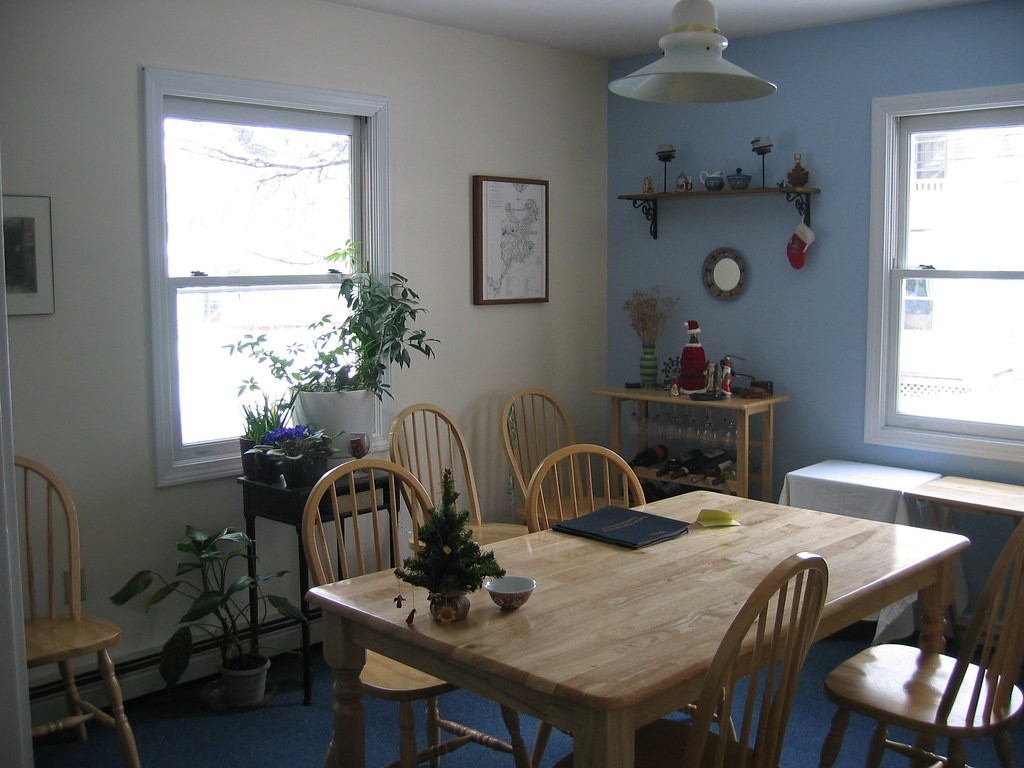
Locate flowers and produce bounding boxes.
[260,423,315,448]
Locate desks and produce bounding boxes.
[903,474,1024,645]
[778,459,969,647]
[236,471,401,706]
[301,489,973,768]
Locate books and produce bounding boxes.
[552,503,692,550]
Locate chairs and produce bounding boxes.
[532,551,830,768]
[818,516,1024,768]
[524,443,737,768]
[389,401,529,561]
[501,388,625,526]
[12,453,141,768]
[301,458,530,768]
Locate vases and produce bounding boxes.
[254,452,280,482]
[639,344,659,389]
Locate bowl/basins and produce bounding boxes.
[482,575,536,609]
[726,168,752,189]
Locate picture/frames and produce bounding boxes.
[2,195,56,317]
[472,174,549,306]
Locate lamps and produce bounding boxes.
[607,0,778,105]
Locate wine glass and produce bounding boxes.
[627,400,737,451]
[347,430,371,478]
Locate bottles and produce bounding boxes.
[627,444,668,469]
[655,448,760,486]
[721,356,733,397]
[788,154,808,187]
[641,176,653,193]
[671,374,679,396]
[679,321,707,394]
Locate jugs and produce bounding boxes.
[699,170,724,190]
[676,171,692,192]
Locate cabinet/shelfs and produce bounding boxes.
[591,385,793,504]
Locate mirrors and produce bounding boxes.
[701,247,748,301]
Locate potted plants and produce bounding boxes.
[109,523,310,711]
[393,467,507,627]
[243,428,348,488]
[238,390,295,481]
[218,238,443,469]
